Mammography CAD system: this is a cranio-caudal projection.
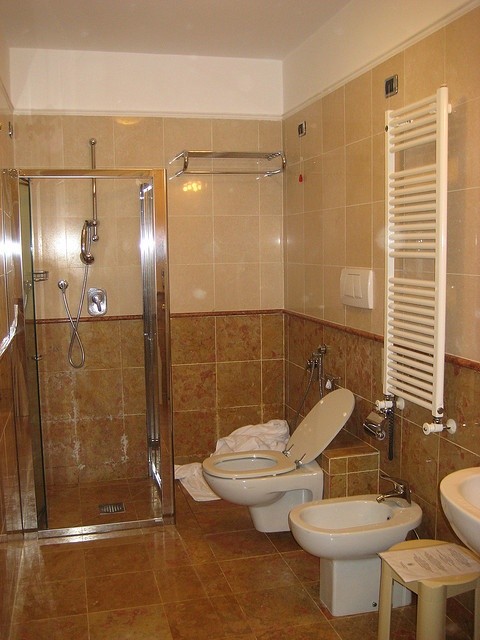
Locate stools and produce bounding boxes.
[377,540,480,640]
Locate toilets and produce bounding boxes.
[202,388,356,533]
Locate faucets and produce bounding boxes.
[377,475,412,510]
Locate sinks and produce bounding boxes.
[288,493,423,617]
[439,467,480,557]
